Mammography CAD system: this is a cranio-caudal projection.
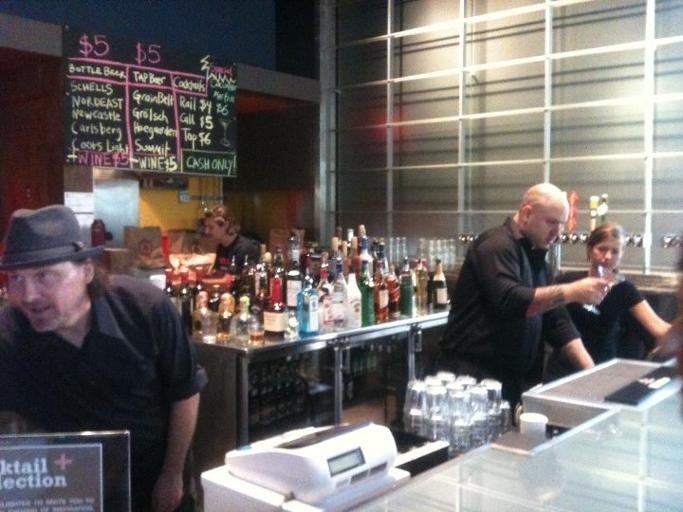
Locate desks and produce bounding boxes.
[185,302,455,512]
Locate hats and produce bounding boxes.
[0,205,103,271]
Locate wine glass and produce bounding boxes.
[582,264,626,315]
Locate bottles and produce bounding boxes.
[589,193,609,232]
[158,224,456,345]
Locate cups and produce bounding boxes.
[402,370,549,454]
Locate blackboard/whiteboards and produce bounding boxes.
[65,31,236,178]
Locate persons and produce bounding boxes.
[430,182,612,426]
[645,255,683,380]
[203,203,260,276]
[539,222,673,386]
[0,203,210,512]
